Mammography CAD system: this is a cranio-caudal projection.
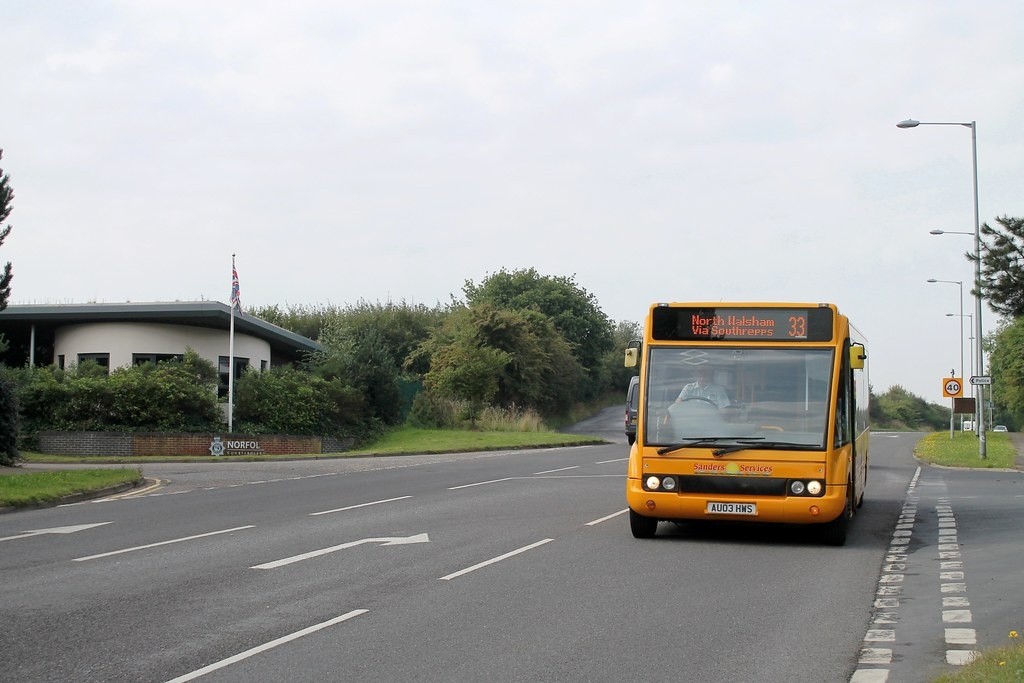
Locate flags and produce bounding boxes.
[229,266,242,316]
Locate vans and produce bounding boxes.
[625,374,641,447]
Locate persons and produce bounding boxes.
[675,365,730,408]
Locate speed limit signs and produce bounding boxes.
[944,380,961,396]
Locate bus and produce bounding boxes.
[625,298,872,543]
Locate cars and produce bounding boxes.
[992,425,1010,433]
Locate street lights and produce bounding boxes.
[895,118,987,460]
[926,277,965,431]
[945,312,975,431]
[928,228,982,439]
